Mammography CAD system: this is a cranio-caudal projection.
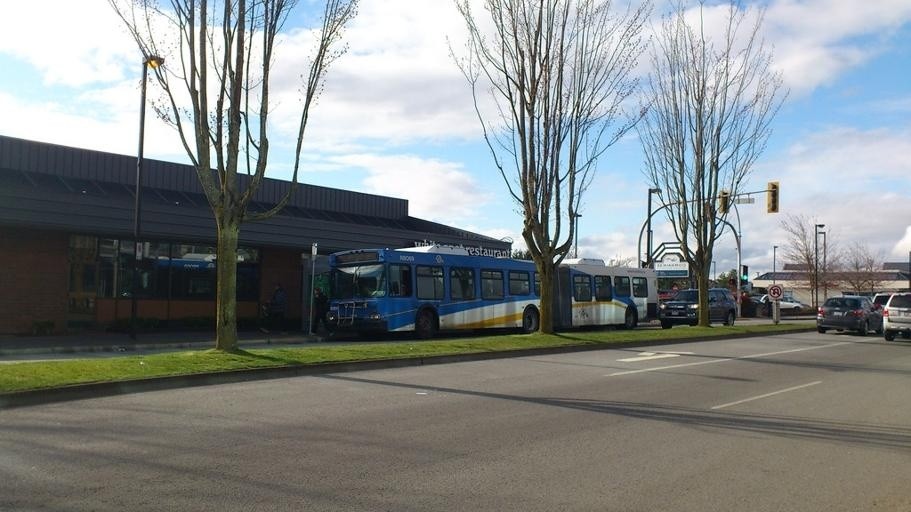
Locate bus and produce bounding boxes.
[89,247,267,336]
[320,243,662,345]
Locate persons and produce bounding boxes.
[261,283,288,334]
[309,288,333,335]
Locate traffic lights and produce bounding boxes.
[740,264,750,287]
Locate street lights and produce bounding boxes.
[711,260,717,282]
[125,51,167,346]
[574,213,584,260]
[646,187,663,267]
[813,222,826,318]
[818,230,829,303]
[772,244,780,285]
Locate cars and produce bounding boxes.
[816,291,911,341]
[656,287,679,301]
[732,293,811,314]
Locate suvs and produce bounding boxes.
[657,286,740,331]
